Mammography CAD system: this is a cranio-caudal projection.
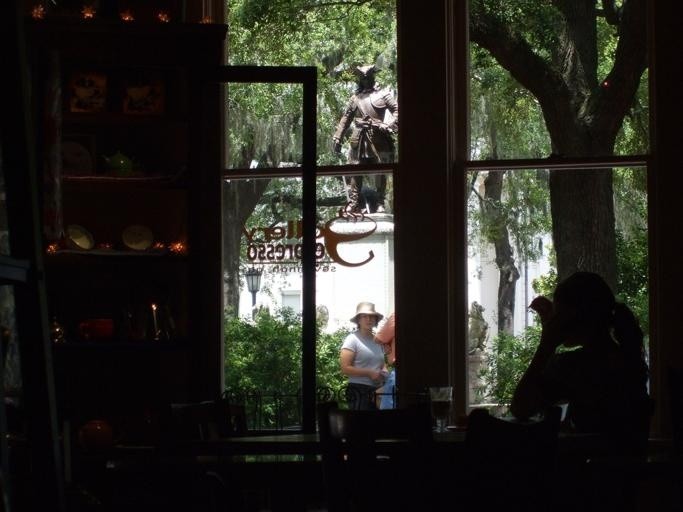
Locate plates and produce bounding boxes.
[120,225,153,251]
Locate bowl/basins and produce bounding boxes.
[65,225,96,251]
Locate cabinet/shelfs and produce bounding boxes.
[40,11,205,464]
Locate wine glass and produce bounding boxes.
[427,386,453,434]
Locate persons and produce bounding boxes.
[508,270,654,512]
[331,64,399,214]
[373,313,396,410]
[339,301,389,411]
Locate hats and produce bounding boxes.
[350,303,383,323]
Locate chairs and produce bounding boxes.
[151,398,657,512]
